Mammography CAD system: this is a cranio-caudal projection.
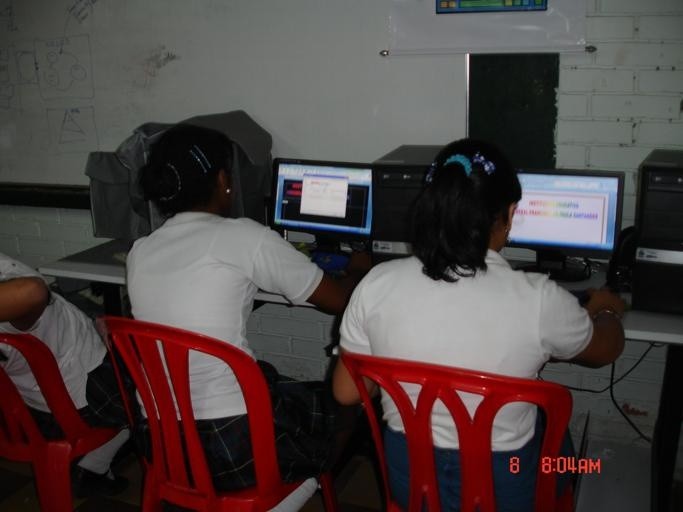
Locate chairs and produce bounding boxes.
[94,315,338,512]
[0,332,121,512]
[337,347,574,511]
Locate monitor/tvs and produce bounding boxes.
[504,169,626,281]
[268,157,372,258]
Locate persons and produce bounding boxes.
[0,252,136,497]
[126,123,359,493]
[331,139,625,512]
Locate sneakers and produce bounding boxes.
[74,466,128,498]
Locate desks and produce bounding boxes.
[36,238,681,512]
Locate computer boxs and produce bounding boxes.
[632,150,683,316]
[372,145,447,262]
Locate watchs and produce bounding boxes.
[591,306,622,321]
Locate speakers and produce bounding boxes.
[604,224,635,293]
